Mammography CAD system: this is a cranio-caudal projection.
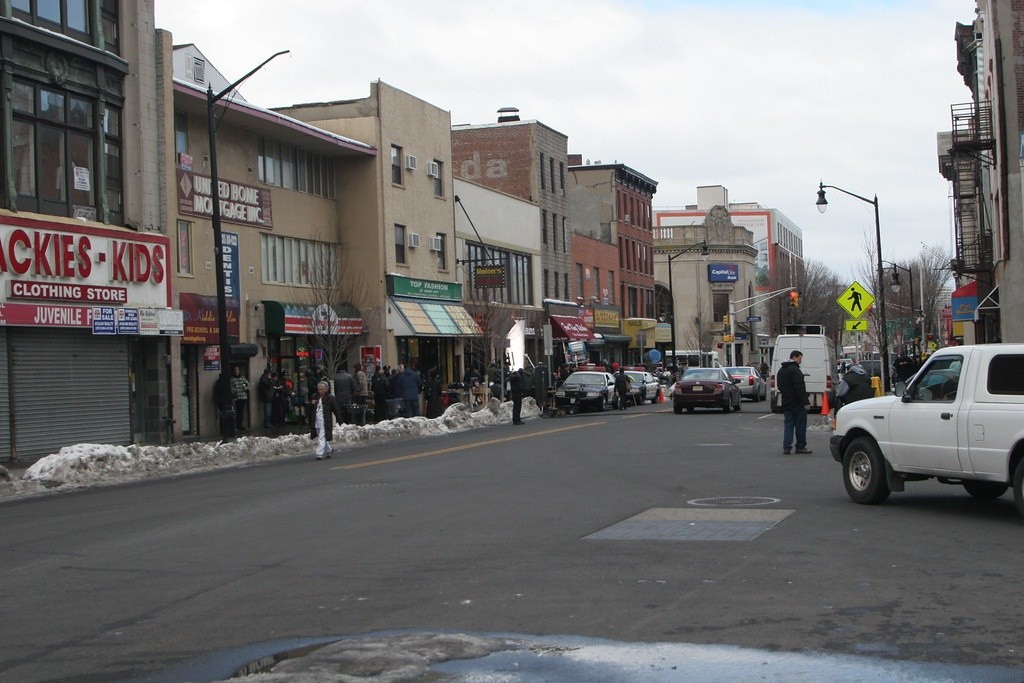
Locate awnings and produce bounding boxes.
[260,300,369,336]
[951,280,979,321]
[386,295,485,337]
[549,315,598,341]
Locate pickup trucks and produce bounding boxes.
[830,344,1024,526]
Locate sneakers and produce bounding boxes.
[795,447,812,454]
[783,448,790,454]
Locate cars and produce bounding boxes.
[556,371,617,412]
[673,368,742,414]
[614,371,660,405]
[725,366,767,403]
[836,346,899,378]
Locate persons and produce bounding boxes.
[532,360,578,411]
[213,365,251,437]
[836,363,875,406]
[927,360,961,401]
[463,362,510,407]
[614,367,631,411]
[777,350,813,454]
[308,381,343,459]
[760,360,769,382]
[891,352,931,388]
[258,362,444,429]
[510,368,525,425]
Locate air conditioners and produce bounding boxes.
[406,155,417,171]
[409,234,420,247]
[427,162,438,178]
[430,238,441,251]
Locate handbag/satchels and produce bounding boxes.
[612,394,618,409]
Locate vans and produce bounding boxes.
[770,334,840,411]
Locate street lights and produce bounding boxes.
[668,239,710,375]
[876,260,925,362]
[816,179,900,393]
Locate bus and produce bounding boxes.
[666,350,724,369]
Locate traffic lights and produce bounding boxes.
[723,314,728,325]
[790,291,798,308]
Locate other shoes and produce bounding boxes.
[513,421,524,425]
[316,455,323,459]
[326,449,334,458]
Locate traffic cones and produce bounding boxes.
[659,390,664,403]
[820,390,830,414]
